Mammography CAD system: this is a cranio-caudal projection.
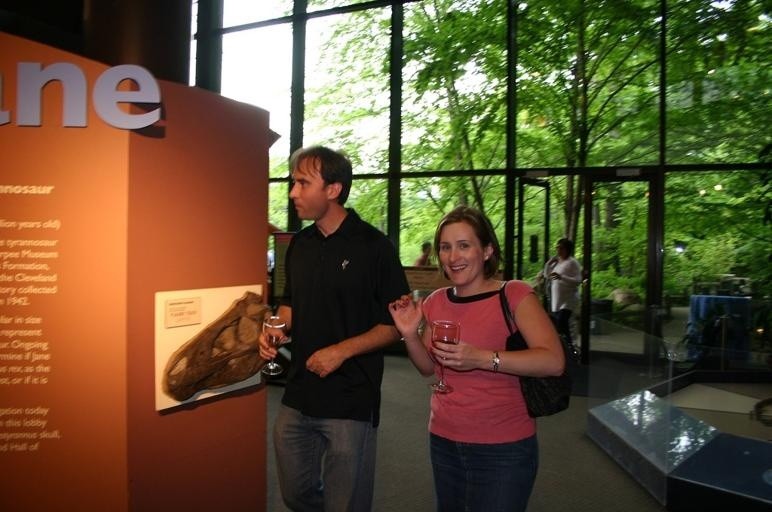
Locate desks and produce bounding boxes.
[688,294,755,370]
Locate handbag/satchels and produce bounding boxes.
[505,308,575,417]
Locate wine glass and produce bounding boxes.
[261,316,287,377]
[427,318,462,394]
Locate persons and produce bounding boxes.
[257,146,415,512]
[388,205,569,511]
[416,241,434,267]
[537,237,583,348]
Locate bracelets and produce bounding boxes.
[490,350,500,373]
